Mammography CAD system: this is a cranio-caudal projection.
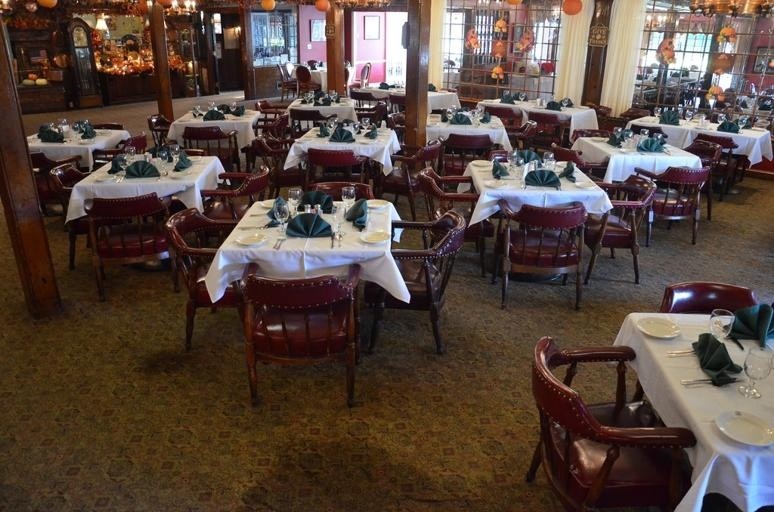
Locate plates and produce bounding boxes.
[259,199,276,208]
[428,92,750,188]
[234,233,266,245]
[367,199,389,210]
[714,408,774,448]
[361,231,392,244]
[638,314,681,340]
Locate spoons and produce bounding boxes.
[330,232,335,248]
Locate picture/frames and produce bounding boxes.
[364,16,380,40]
[752,46,774,75]
[310,19,328,42]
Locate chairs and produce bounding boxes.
[524,334,696,512]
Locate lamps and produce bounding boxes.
[690,0,774,19]
[644,15,667,29]
[162,0,199,18]
[95,13,110,31]
[234,26,241,40]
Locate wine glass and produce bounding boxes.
[273,200,291,238]
[330,205,347,238]
[341,186,355,210]
[738,346,774,398]
[710,309,736,344]
[32,59,408,182]
[287,187,302,216]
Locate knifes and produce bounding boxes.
[681,375,746,386]
[718,326,744,351]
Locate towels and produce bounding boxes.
[730,304,774,347]
